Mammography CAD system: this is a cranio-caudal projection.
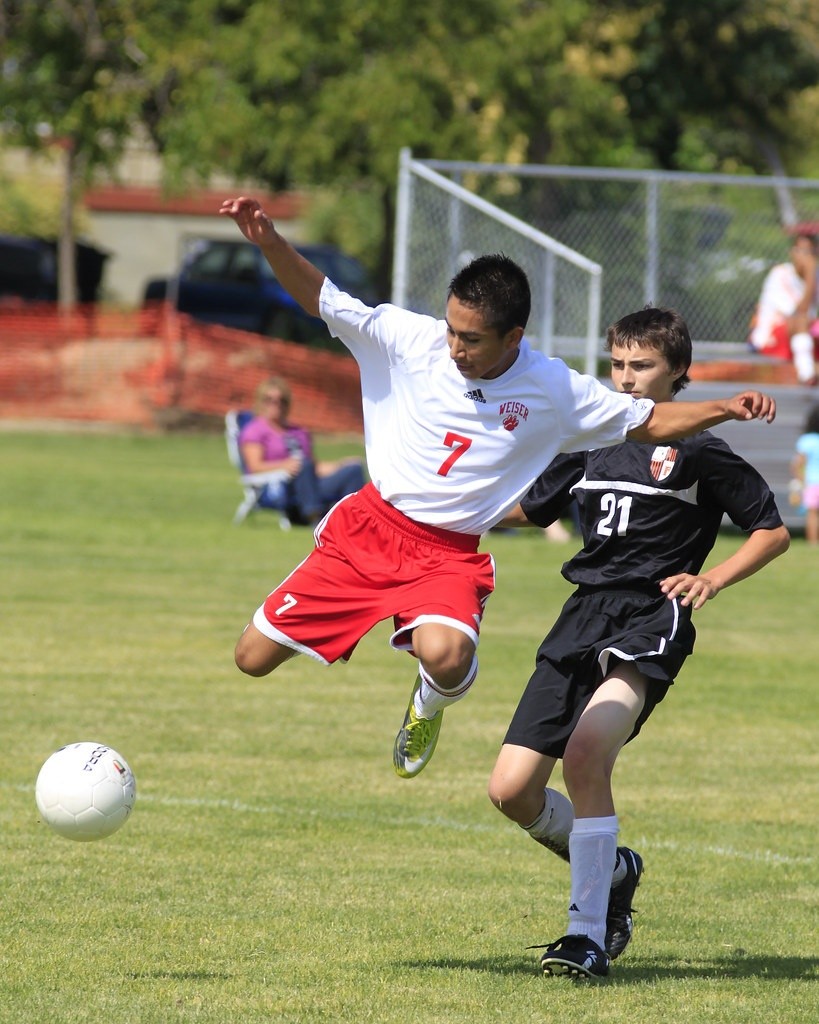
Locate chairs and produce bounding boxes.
[224,409,293,531]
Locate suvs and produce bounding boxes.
[178,240,381,351]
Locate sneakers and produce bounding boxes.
[604,847,644,961]
[393,674,444,779]
[525,933,611,982]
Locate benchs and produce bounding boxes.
[687,358,819,387]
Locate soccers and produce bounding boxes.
[35,741,134,843]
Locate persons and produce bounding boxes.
[789,404,819,544]
[219,196,776,780]
[487,306,792,983]
[241,375,366,529]
[752,232,818,389]
